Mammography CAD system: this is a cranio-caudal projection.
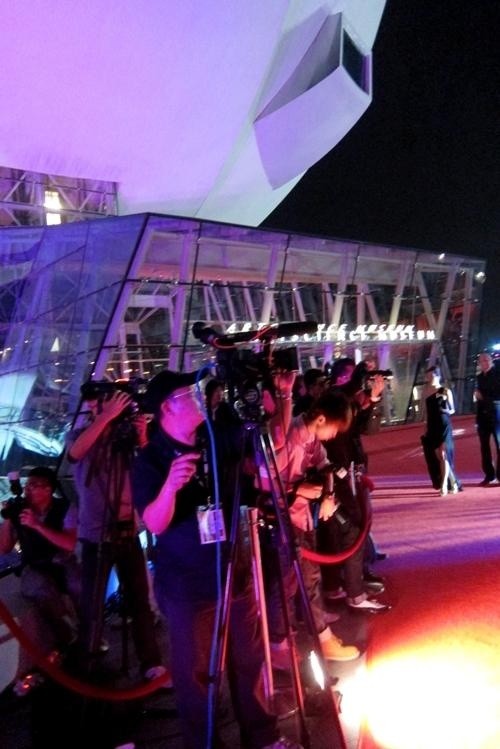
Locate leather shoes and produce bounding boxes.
[362,571,383,583]
[347,598,392,617]
[480,480,489,486]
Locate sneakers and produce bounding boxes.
[262,734,301,748]
[363,581,385,595]
[144,665,173,690]
[321,611,340,624]
[376,554,387,561]
[99,638,109,652]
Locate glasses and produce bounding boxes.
[25,483,41,491]
[171,388,196,398]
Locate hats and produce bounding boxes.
[143,368,208,415]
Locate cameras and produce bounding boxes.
[306,464,338,496]
[352,361,394,385]
[0,471,30,520]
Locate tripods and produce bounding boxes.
[86,431,130,670]
[206,422,348,749]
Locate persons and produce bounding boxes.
[60,357,393,749]
[419,365,463,499]
[471,352,500,487]
[0,465,111,657]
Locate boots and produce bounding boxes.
[317,625,360,661]
[269,638,301,671]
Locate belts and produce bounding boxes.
[493,401,500,405]
